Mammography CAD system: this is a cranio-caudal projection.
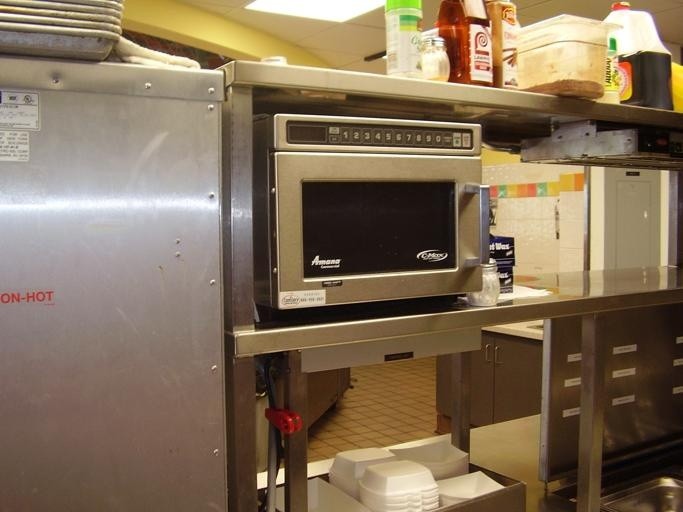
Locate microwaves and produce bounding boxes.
[255,113,490,321]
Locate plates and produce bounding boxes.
[327,439,506,512]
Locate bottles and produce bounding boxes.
[594,35,619,105]
[385,0,520,91]
[467,261,501,307]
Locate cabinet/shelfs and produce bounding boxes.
[219,59,682,510]
